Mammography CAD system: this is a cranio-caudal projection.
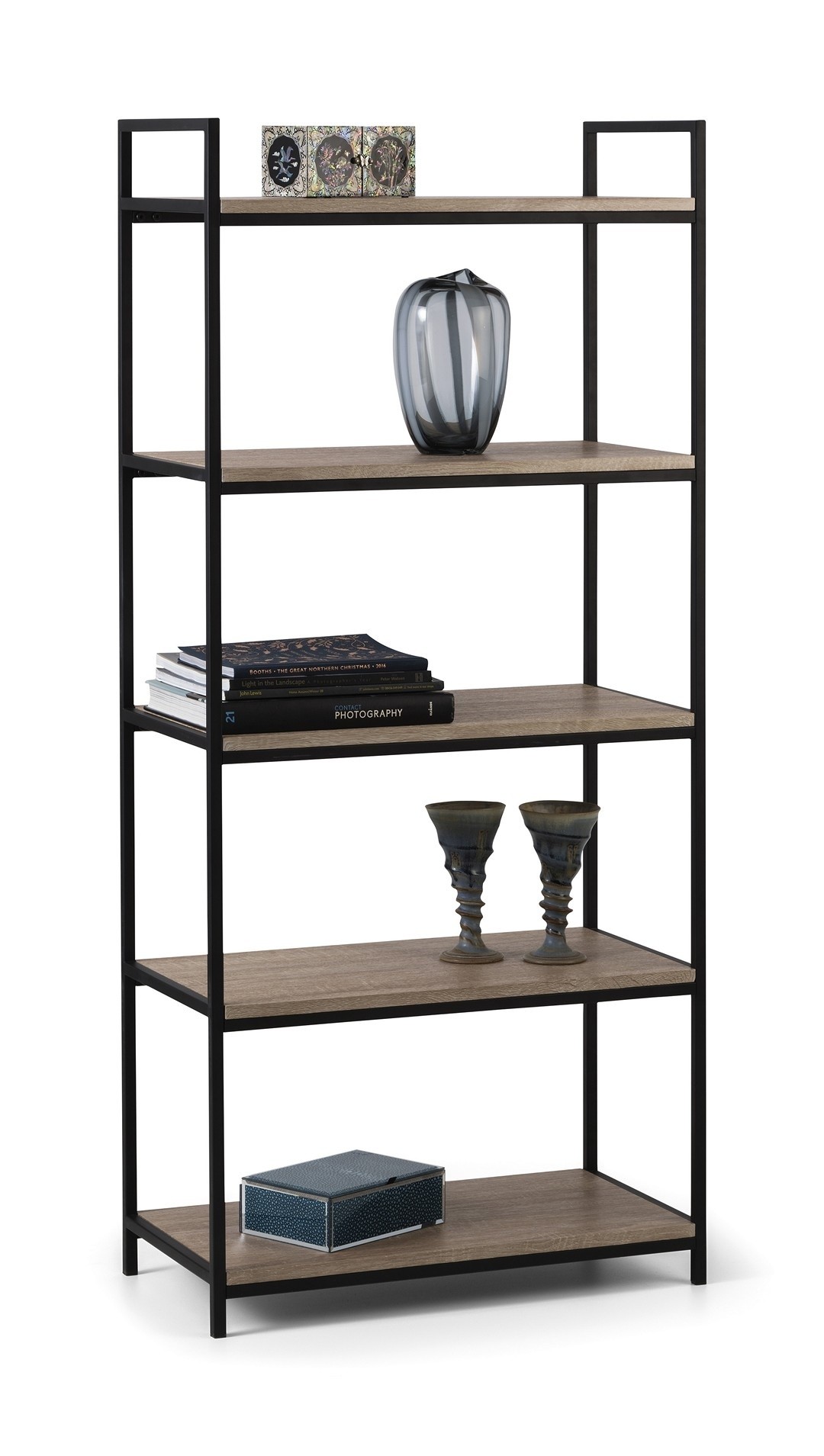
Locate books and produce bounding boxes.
[143,635,455,735]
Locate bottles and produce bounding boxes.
[392,271,511,458]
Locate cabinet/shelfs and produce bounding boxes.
[122,118,707,1338]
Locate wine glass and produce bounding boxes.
[518,801,601,965]
[424,801,504,964]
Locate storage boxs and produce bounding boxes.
[239,1150,446,1252]
[260,126,416,198]
